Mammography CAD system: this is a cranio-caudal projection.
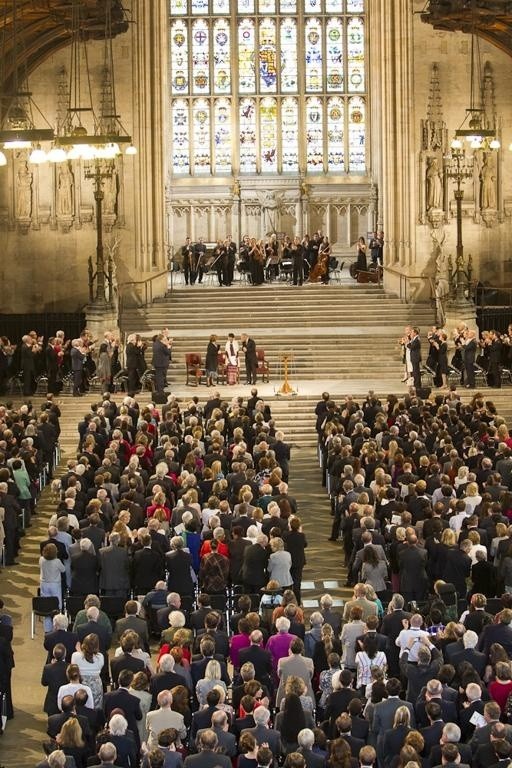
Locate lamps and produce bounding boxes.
[451,34,512,150]
[0,27,138,167]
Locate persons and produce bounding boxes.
[314,387,512,603]
[180,231,383,286]
[0,580,512,767]
[398,322,512,389]
[1,392,310,632]
[1,326,258,400]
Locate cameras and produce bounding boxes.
[413,637,420,642]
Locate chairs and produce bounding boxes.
[176,253,351,283]
[421,364,512,389]
[185,353,206,387]
[218,353,240,385]
[1,369,158,394]
[30,597,292,635]
[256,349,270,383]
[0,444,60,566]
